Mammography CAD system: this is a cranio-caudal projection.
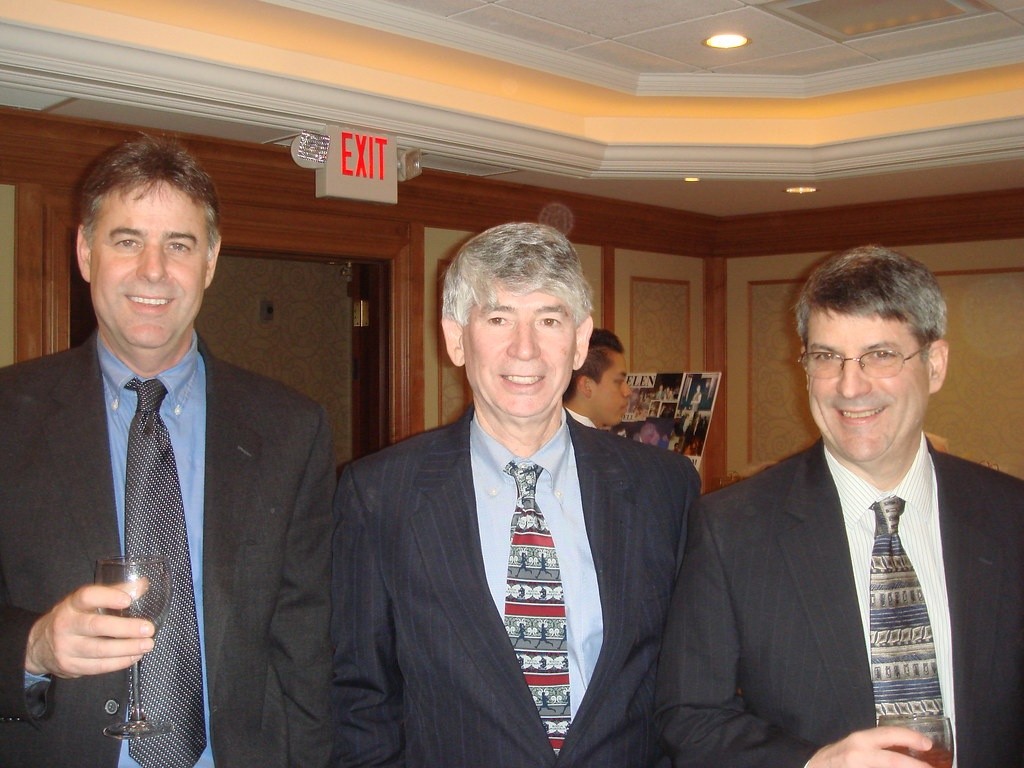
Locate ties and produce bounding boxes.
[867,495,945,749]
[124,377,208,768]
[505,460,571,757]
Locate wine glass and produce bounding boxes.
[93,554,173,739]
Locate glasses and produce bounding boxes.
[795,342,931,379]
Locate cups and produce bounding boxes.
[879,714,955,768]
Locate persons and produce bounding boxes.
[329,220,702,768]
[563,327,634,431]
[657,242,1023,768]
[0,130,338,768]
[619,375,709,456]
